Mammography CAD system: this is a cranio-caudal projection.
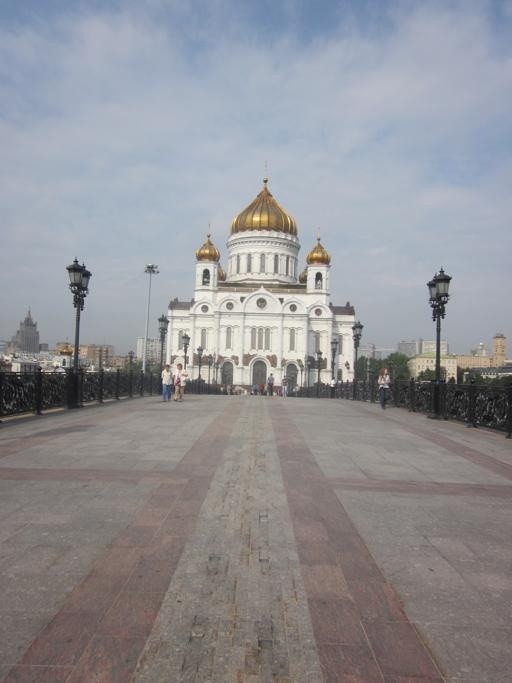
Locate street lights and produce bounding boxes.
[158,315,170,368]
[299,350,322,385]
[143,264,160,372]
[66,258,92,370]
[351,322,363,381]
[182,333,220,382]
[330,339,339,377]
[427,267,452,384]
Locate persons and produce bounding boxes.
[376,367,392,409]
[160,364,174,401]
[217,382,283,397]
[267,373,275,396]
[172,363,188,401]
[329,376,337,398]
